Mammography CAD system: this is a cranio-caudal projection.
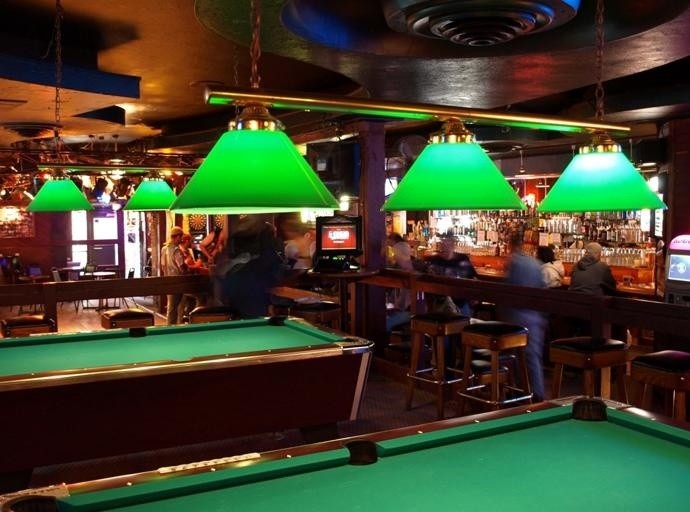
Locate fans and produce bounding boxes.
[384,130,433,190]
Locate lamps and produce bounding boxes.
[166,2,669,224]
[17,2,196,213]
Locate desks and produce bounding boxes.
[1,310,378,470]
[1,390,688,510]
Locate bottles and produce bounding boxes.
[406,204,656,269]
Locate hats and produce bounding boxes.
[170,226,184,235]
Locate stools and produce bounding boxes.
[630,346,688,422]
[454,317,534,414]
[545,333,633,404]
[403,306,465,420]
[188,304,237,322]
[0,312,56,339]
[98,304,154,330]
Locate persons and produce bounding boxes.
[495,236,549,402]
[568,242,617,338]
[535,246,568,371]
[160,220,413,325]
[420,237,487,380]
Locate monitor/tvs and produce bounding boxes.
[307,141,361,196]
[316,217,362,255]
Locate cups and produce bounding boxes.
[622,275,635,288]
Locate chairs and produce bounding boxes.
[0,252,143,315]
